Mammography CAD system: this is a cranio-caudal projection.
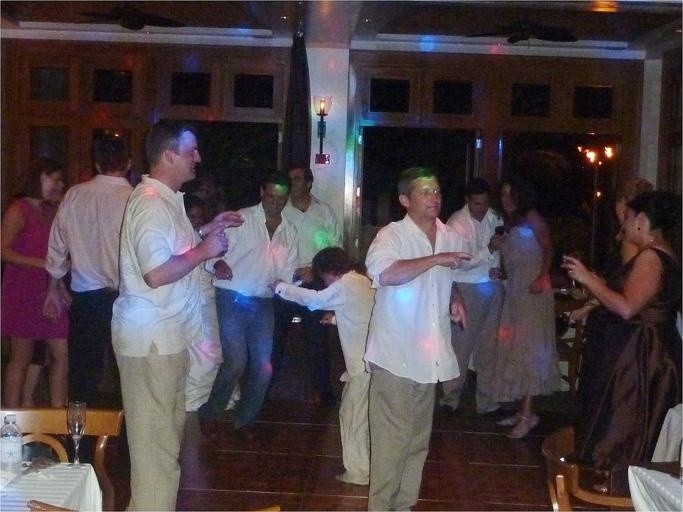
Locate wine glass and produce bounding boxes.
[560,249,580,294]
[62,399,88,467]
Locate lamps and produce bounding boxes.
[313,96,333,164]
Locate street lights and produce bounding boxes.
[580,144,617,269]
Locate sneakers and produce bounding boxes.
[308,385,337,408]
[439,403,508,422]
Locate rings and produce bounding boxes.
[45,312,48,317]
[63,304,68,307]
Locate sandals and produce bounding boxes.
[497,410,540,439]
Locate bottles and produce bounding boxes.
[0,413,25,479]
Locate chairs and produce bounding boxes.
[541,425,680,512]
[0,407,123,512]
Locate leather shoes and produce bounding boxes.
[234,417,259,443]
[199,405,228,443]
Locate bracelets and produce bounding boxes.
[196,225,205,240]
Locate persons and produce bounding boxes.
[185,151,380,484]
[485,170,557,440]
[439,178,513,426]
[559,185,682,497]
[1,154,70,412]
[569,176,650,328]
[111,116,245,510]
[42,126,133,443]
[363,165,502,511]
